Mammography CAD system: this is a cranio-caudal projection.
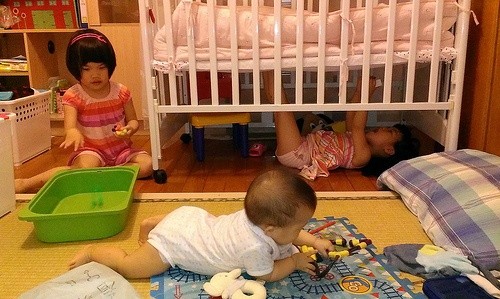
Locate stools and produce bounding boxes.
[188,98,251,161]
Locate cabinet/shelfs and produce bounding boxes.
[0,0,148,130]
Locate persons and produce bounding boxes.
[14,28,153,193]
[69,169,334,284]
[260,71,420,180]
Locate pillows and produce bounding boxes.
[376,149,500,272]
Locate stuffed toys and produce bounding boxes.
[203,269,267,299]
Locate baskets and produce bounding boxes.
[0,90,53,167]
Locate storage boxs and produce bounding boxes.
[0,89,52,166]
[18,166,140,243]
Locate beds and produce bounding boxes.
[138,0,472,185]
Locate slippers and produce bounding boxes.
[249,142,271,157]
